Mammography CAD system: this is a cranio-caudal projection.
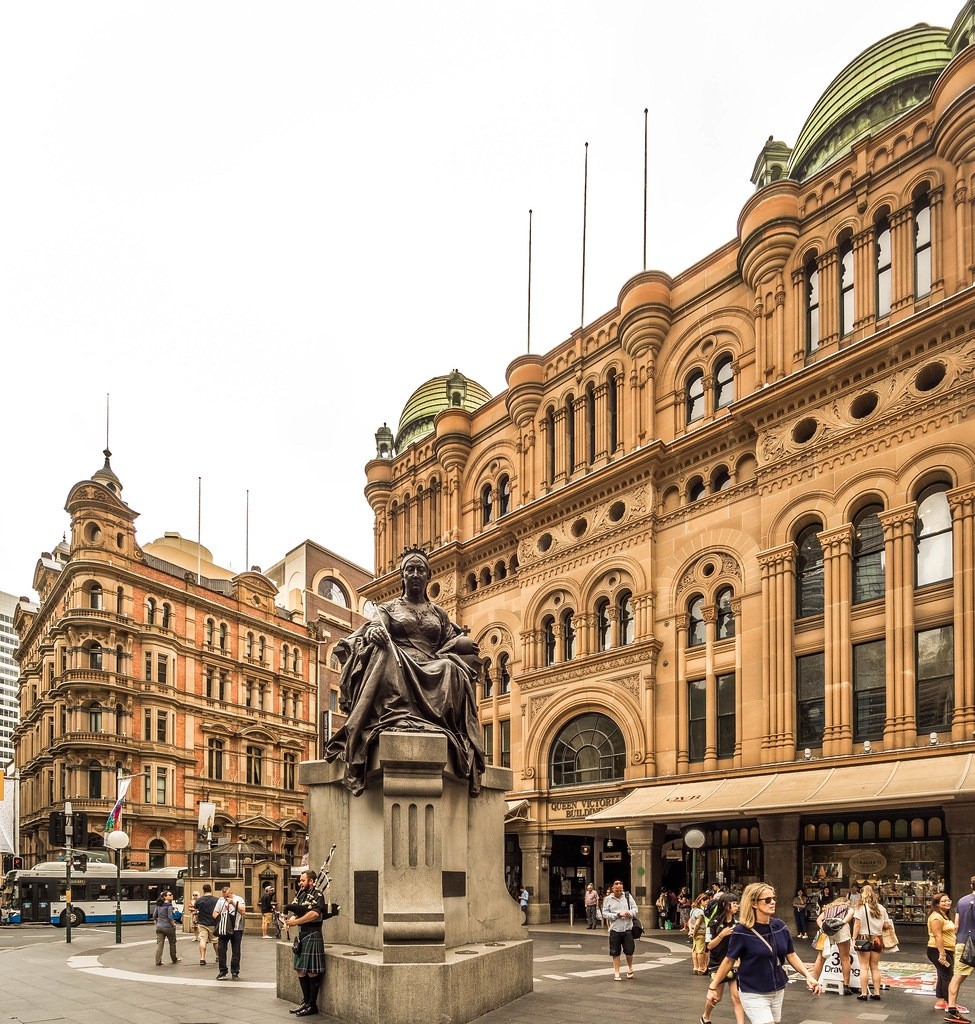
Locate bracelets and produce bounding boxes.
[709,988,716,990]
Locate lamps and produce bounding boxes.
[928,731,940,745]
[607,827,614,849]
[803,748,812,761]
[863,741,873,754]
[580,828,591,856]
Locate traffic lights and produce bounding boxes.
[124,856,131,869]
[14,856,23,870]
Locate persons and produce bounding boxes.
[154,892,181,965]
[189,884,219,965]
[807,898,855,995]
[700,893,744,1024]
[852,886,889,1000]
[324,543,485,797]
[212,887,245,980]
[285,870,325,1016]
[261,887,276,938]
[603,881,639,980]
[793,886,832,938]
[846,884,860,937]
[944,877,975,1024]
[908,882,915,897]
[707,883,822,1024]
[659,883,725,974]
[519,887,528,925]
[584,883,612,930]
[927,893,970,1013]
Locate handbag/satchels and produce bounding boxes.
[293,936,303,957]
[822,936,831,958]
[632,916,645,939]
[708,964,738,984]
[853,939,872,951]
[816,893,822,916]
[213,899,239,937]
[881,919,900,948]
[596,908,603,921]
[959,933,975,969]
[656,894,665,907]
[821,919,842,937]
[880,943,900,954]
[664,919,671,930]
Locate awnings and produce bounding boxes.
[504,800,530,823]
[585,754,975,821]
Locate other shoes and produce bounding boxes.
[156,935,272,981]
[699,1016,712,1024]
[586,925,706,981]
[798,934,808,939]
[844,984,971,1023]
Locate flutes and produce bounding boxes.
[283,844,341,940]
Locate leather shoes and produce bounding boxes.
[290,999,318,1016]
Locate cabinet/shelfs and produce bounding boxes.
[882,894,932,924]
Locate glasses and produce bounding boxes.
[753,896,777,904]
[615,882,622,886]
[940,900,952,904]
[166,894,173,896]
[193,895,197,897]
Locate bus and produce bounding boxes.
[0,858,310,928]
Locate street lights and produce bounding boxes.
[107,830,130,944]
[115,771,150,830]
[684,830,705,905]
